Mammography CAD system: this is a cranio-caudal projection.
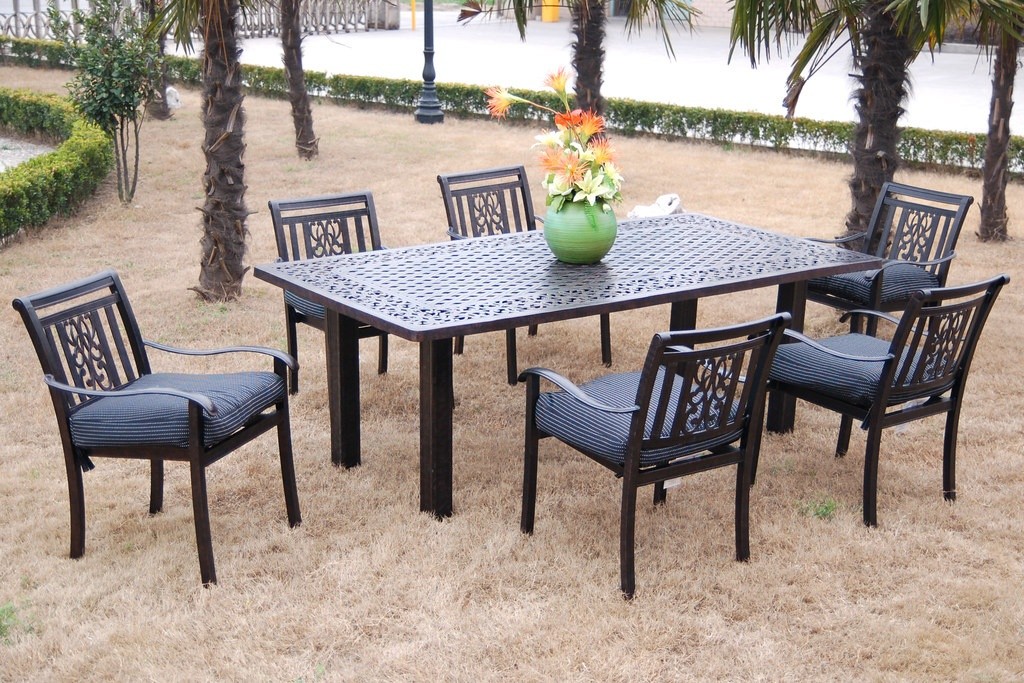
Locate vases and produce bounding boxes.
[544,201,618,267]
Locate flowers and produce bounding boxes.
[486,67,626,214]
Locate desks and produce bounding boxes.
[254,213,888,524]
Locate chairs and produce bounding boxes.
[436,165,612,386]
[12,270,303,590]
[803,181,976,340]
[746,274,1011,525]
[516,312,795,603]
[266,190,391,394]
